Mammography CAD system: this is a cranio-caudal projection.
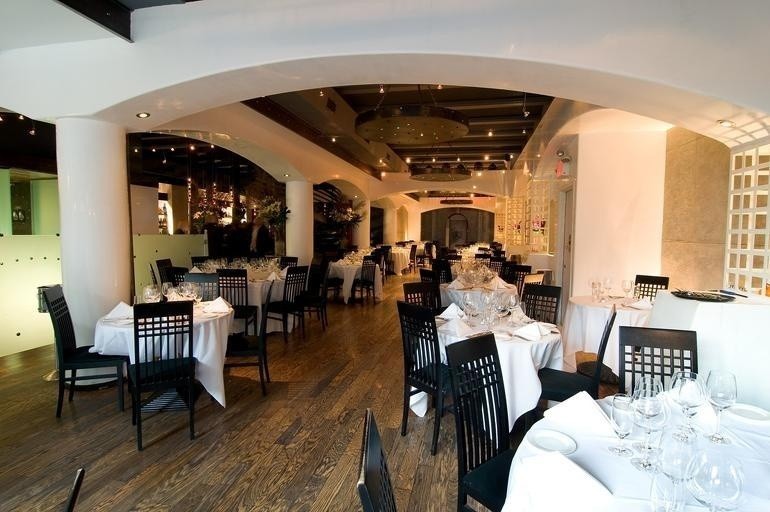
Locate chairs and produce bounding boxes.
[356,408,396,512]
[395,232,564,327]
[64,468,84,512]
[211,279,275,402]
[634,274,669,300]
[42,284,129,417]
[522,304,616,439]
[151,241,392,342]
[445,333,520,512]
[127,300,194,450]
[619,326,699,398]
[401,301,484,455]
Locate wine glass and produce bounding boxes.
[457,291,526,329]
[607,370,743,511]
[202,255,279,280]
[134,281,204,308]
[589,278,632,308]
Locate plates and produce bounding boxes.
[729,402,770,424]
[527,428,576,454]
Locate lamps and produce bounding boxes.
[356,86,470,145]
